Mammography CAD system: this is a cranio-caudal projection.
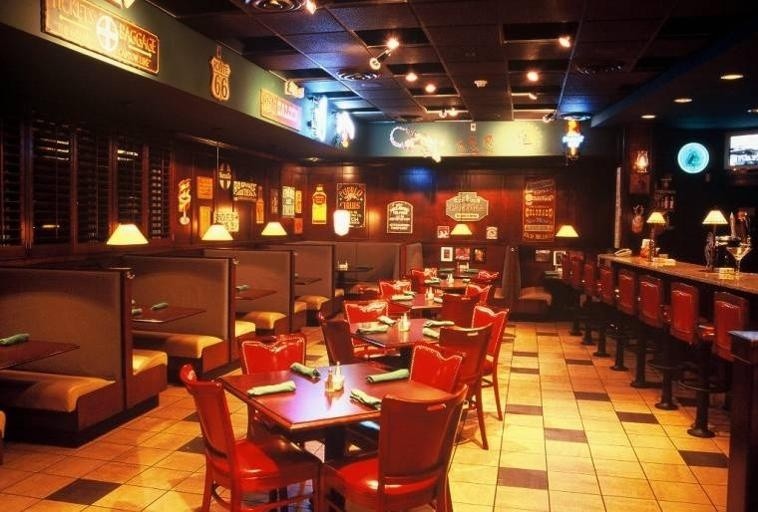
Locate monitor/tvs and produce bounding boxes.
[723,130,758,170]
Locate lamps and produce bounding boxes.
[646,211,666,238]
[260,160,288,236]
[703,209,729,246]
[556,159,579,238]
[106,135,149,245]
[201,144,233,241]
[450,182,472,235]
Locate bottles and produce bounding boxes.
[398,314,409,331]
[333,361,344,391]
[648,230,656,261]
[325,368,334,393]
[447,273,454,283]
[655,193,675,209]
[663,176,669,191]
[256,186,264,224]
[310,184,328,225]
[425,287,434,300]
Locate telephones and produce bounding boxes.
[614,248,632,257]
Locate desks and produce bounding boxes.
[727,330,758,512]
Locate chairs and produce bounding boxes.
[180,269,509,511]
[563,253,748,435]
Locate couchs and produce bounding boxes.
[405,243,553,316]
[1,240,403,441]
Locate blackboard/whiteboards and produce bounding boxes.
[336,183,366,229]
[386,201,413,234]
[522,176,557,242]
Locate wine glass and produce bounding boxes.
[179,194,192,226]
[726,245,751,278]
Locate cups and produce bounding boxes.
[359,319,370,328]
[402,288,410,296]
